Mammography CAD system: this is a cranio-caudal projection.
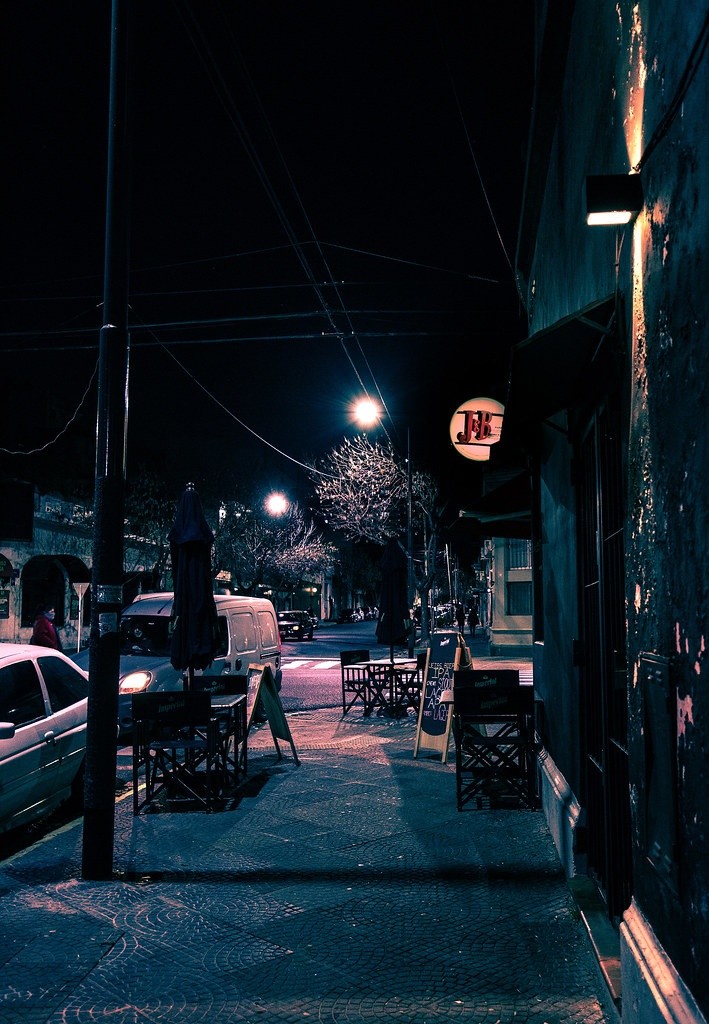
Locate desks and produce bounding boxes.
[355,658,417,716]
[151,693,246,792]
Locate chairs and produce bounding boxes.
[452,669,534,812]
[131,675,248,811]
[340,649,427,719]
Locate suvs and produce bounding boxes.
[275,610,314,641]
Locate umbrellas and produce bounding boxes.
[376,537,412,705]
[168,482,225,692]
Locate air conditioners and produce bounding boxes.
[484,539,491,555]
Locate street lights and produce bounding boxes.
[353,400,415,660]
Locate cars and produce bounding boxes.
[414,603,456,627]
[344,605,379,622]
[0,643,120,832]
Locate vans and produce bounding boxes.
[70,594,282,738]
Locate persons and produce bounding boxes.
[32,603,62,652]
[458,606,480,635]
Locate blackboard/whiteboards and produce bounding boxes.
[246,663,293,741]
[415,632,474,752]
